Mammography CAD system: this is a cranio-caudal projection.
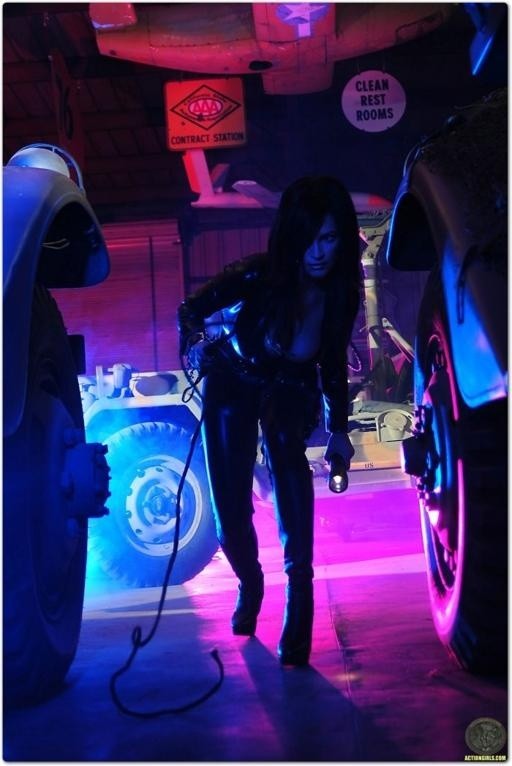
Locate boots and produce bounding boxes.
[277,579,319,666]
[232,567,263,636]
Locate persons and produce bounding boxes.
[170,176,369,672]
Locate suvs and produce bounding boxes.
[73,361,271,594]
[382,3,508,676]
